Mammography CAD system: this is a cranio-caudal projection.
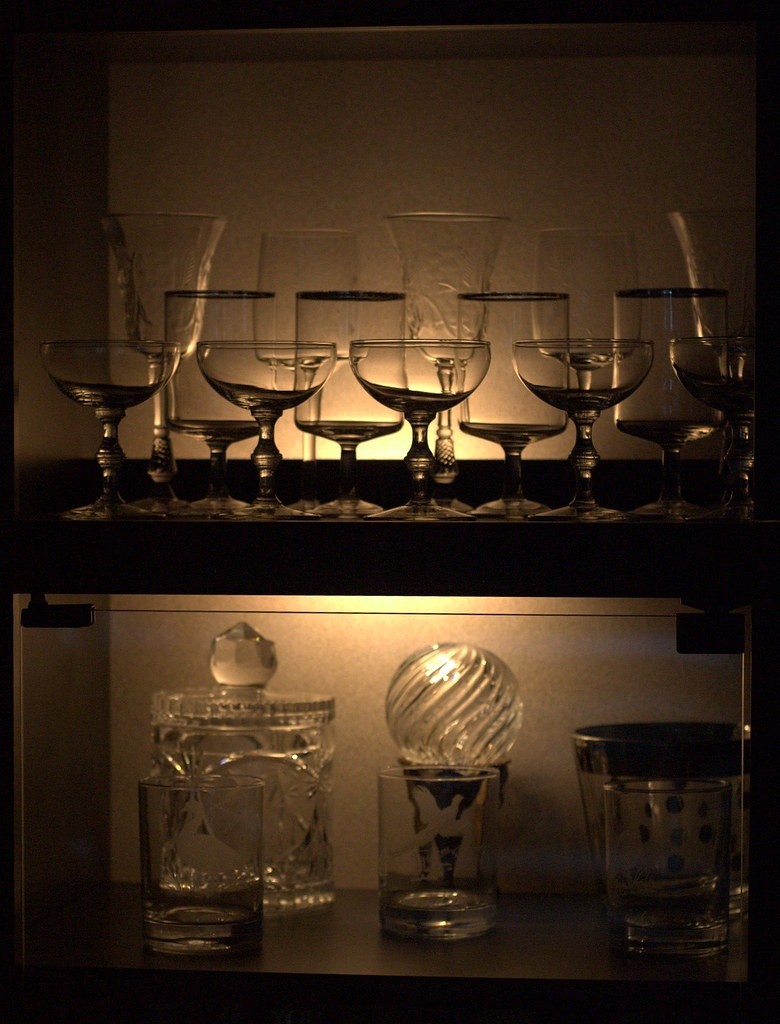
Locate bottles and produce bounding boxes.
[151,622,336,918]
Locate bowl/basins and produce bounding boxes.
[571,722,749,918]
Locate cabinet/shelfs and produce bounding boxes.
[12,19,761,982]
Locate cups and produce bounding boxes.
[604,777,732,956]
[138,774,262,955]
[376,766,499,942]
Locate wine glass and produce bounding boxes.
[671,336,755,518]
[164,290,275,512]
[98,211,226,515]
[386,214,510,514]
[295,289,404,515]
[40,340,180,521]
[529,229,642,391]
[613,286,728,517]
[511,339,654,519]
[197,340,336,521]
[458,293,570,518]
[351,339,492,522]
[252,230,360,515]
